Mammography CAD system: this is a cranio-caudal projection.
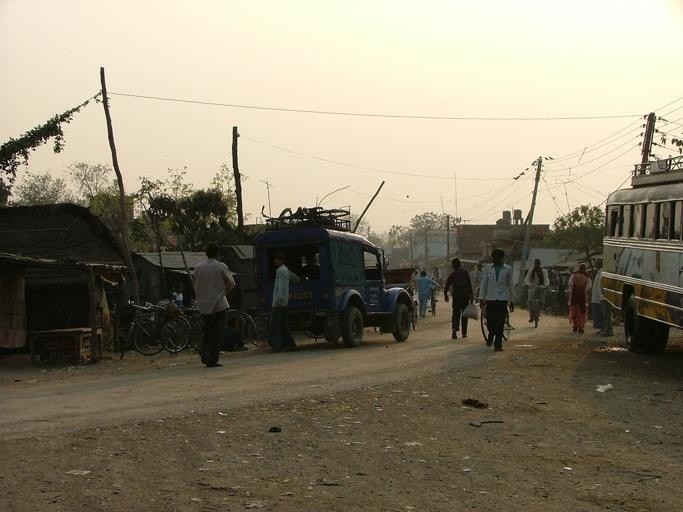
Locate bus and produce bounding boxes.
[598,155,683,353]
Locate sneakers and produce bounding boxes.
[201,354,223,367]
[494,343,505,352]
[487,332,494,347]
[571,325,585,334]
[451,331,467,340]
[268,344,297,354]
[598,329,613,337]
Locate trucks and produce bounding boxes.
[250,217,415,349]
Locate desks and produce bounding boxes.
[28,326,104,365]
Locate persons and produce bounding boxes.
[414,270,440,317]
[566,264,591,334]
[524,258,549,321]
[590,257,614,336]
[443,259,473,338]
[478,249,514,350]
[191,242,235,365]
[265,250,302,351]
[220,315,249,349]
[171,282,183,309]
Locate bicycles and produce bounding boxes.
[116,296,257,363]
[375,286,417,334]
[426,287,443,317]
[529,284,548,327]
[476,287,515,343]
[259,203,351,228]
